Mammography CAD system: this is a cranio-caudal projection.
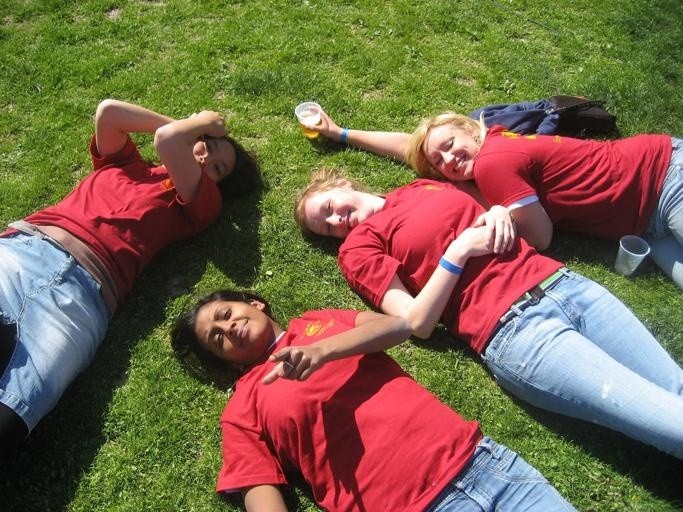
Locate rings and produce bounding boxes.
[285,361,294,369]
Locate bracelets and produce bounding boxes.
[341,128,348,145]
[438,258,464,275]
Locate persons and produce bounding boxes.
[172,290,581,512]
[293,166,683,461]
[0,100,258,474]
[308,99,683,290]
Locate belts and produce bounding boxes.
[489,265,567,344]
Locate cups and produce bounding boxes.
[294,101,321,140]
[612,235,649,278]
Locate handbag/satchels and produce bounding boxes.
[542,95,616,131]
